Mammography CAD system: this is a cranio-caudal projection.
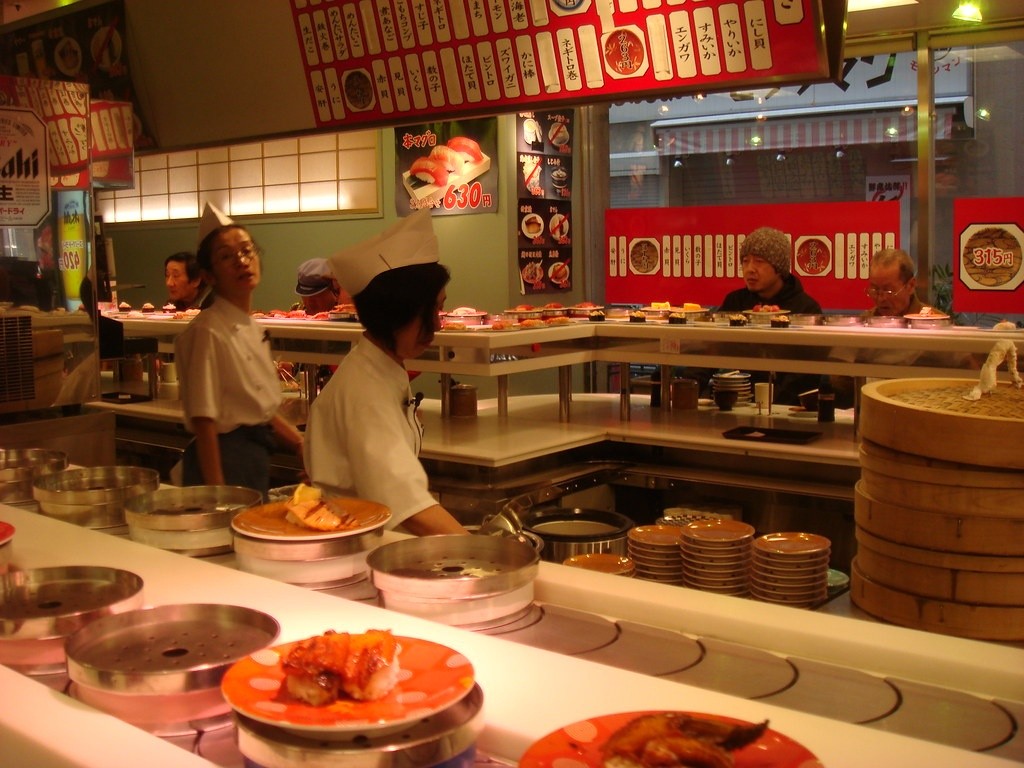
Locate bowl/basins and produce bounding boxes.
[714,390,739,410]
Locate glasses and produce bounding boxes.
[211,244,258,269]
[864,272,917,298]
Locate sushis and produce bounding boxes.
[440,301,791,328]
[603,710,741,768]
[254,307,329,320]
[409,136,485,188]
[281,629,403,706]
[285,483,359,531]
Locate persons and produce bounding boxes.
[717,225,838,411]
[47,340,97,407]
[825,247,980,410]
[299,208,477,541]
[167,201,305,503]
[163,251,215,310]
[286,257,343,396]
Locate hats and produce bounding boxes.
[295,258,335,296]
[197,202,238,247]
[328,207,440,299]
[740,227,791,278]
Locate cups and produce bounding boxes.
[754,382,773,406]
[56,190,89,302]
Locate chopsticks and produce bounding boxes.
[273,360,300,388]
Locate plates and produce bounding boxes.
[99,301,543,335]
[668,308,710,313]
[540,307,569,311]
[520,212,569,285]
[743,310,791,315]
[640,307,674,312]
[0,523,16,547]
[562,517,832,609]
[231,495,396,541]
[710,372,754,408]
[519,708,826,768]
[219,631,474,741]
[903,313,950,319]
[568,305,605,310]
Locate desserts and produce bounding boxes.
[119,301,176,314]
